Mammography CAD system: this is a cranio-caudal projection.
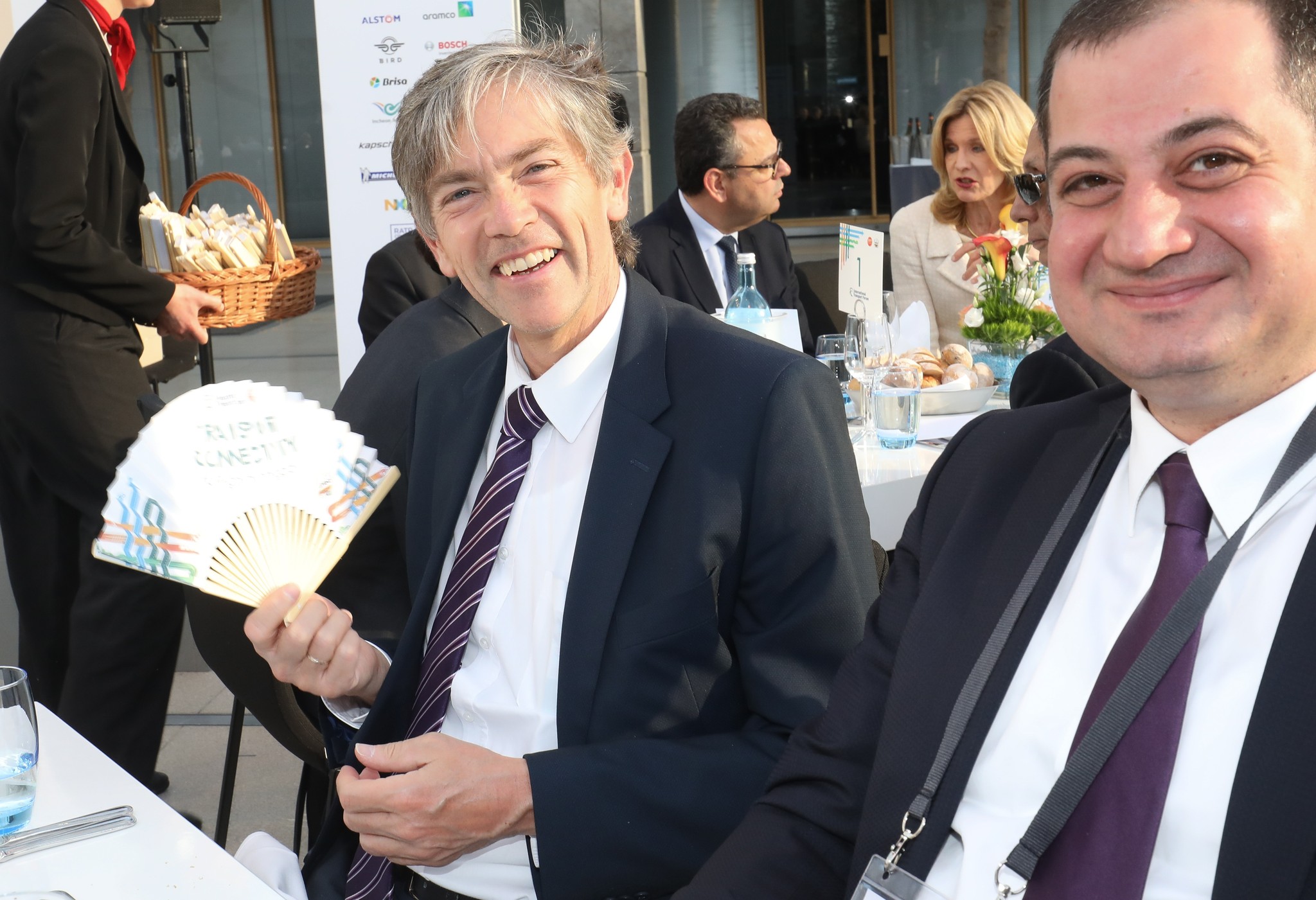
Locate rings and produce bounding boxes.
[306,653,330,667]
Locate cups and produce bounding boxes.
[815,334,860,426]
[882,291,901,350]
[0,665,39,837]
[869,367,921,449]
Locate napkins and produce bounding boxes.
[234,831,308,900]
[887,301,931,357]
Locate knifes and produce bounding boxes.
[0,805,133,847]
[0,814,137,863]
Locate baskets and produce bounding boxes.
[144,171,323,328]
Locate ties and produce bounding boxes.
[344,381,548,900]
[717,235,740,293]
[81,0,137,91]
[1021,453,1213,900]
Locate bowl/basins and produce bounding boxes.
[843,380,1000,415]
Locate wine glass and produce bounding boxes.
[843,313,893,447]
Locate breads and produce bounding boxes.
[845,344,994,391]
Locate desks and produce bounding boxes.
[0,700,284,900]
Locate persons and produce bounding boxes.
[1,0,227,834]
[627,92,849,384]
[358,228,459,353]
[1010,115,1055,268]
[884,79,1035,365]
[849,1,1316,900]
[241,39,877,900]
[246,42,884,900]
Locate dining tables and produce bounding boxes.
[847,395,1010,550]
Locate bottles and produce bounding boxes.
[724,253,771,321]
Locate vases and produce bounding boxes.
[969,338,1043,399]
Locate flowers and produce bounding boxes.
[961,203,1064,358]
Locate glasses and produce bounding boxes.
[1012,170,1047,205]
[721,139,784,180]
